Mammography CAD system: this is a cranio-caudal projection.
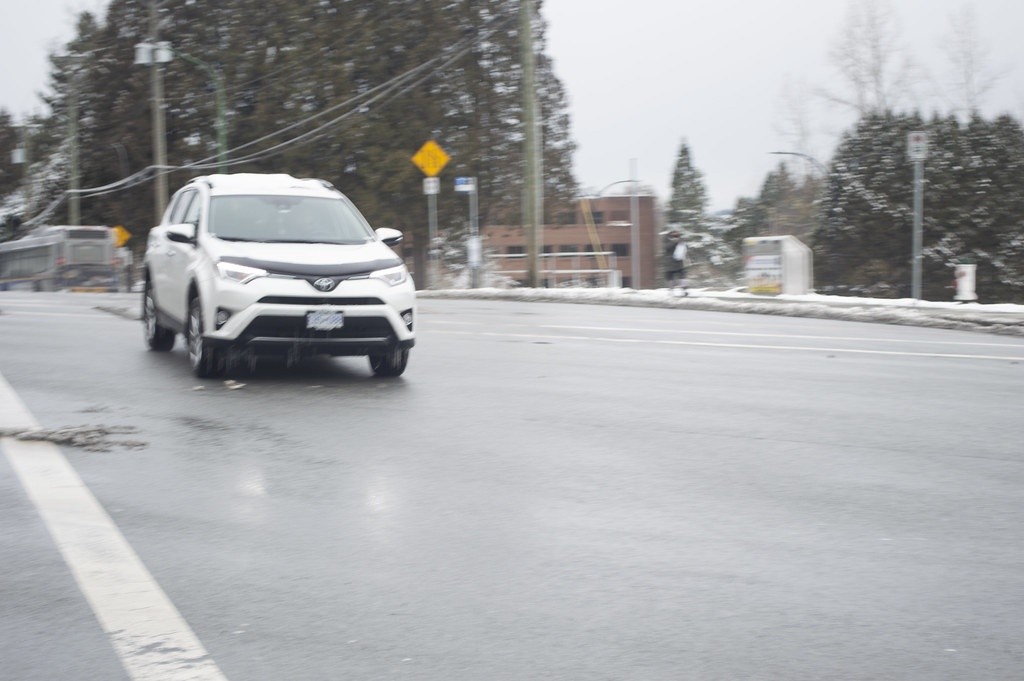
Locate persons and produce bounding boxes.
[659,231,688,289]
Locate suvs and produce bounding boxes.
[142,173,417,379]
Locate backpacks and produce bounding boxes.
[673,242,688,261]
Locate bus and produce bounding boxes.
[0,225,119,292]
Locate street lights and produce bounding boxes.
[133,40,228,175]
[32,77,79,225]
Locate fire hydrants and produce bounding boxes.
[944,256,979,304]
